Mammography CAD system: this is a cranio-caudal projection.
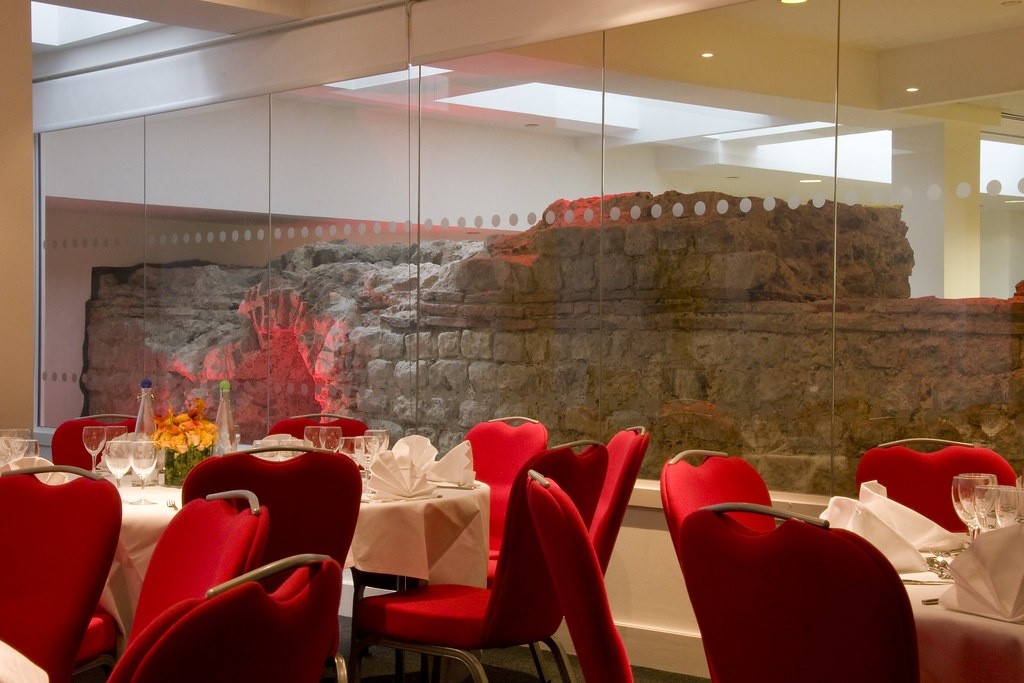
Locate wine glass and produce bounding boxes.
[354,429,390,499]
[82,426,106,477]
[105,440,132,504]
[129,441,159,505]
[951,472,997,548]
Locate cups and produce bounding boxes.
[973,485,1018,533]
[0,428,40,468]
[252,439,304,458]
[1015,473,1024,524]
[303,426,365,467]
[995,489,1024,528]
[235,425,241,445]
[105,426,128,441]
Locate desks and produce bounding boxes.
[898,533,1024,683]
[100,474,491,683]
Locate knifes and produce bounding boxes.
[901,580,953,586]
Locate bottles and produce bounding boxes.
[132,378,159,486]
[212,380,237,457]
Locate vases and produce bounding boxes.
[163,446,214,485]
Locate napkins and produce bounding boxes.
[97,432,148,474]
[938,525,1024,624]
[263,434,303,443]
[0,457,83,486]
[860,480,963,551]
[820,496,928,574]
[392,434,438,477]
[368,451,443,503]
[429,440,481,487]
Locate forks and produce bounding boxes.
[929,549,965,556]
[928,567,955,580]
[167,499,179,511]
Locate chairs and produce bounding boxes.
[126,490,270,648]
[855,439,1017,532]
[50,413,143,472]
[0,464,123,683]
[363,416,652,683]
[107,554,343,683]
[181,444,363,683]
[678,501,919,683]
[72,604,118,675]
[661,449,777,555]
[268,414,368,453]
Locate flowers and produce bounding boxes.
[149,396,219,485]
